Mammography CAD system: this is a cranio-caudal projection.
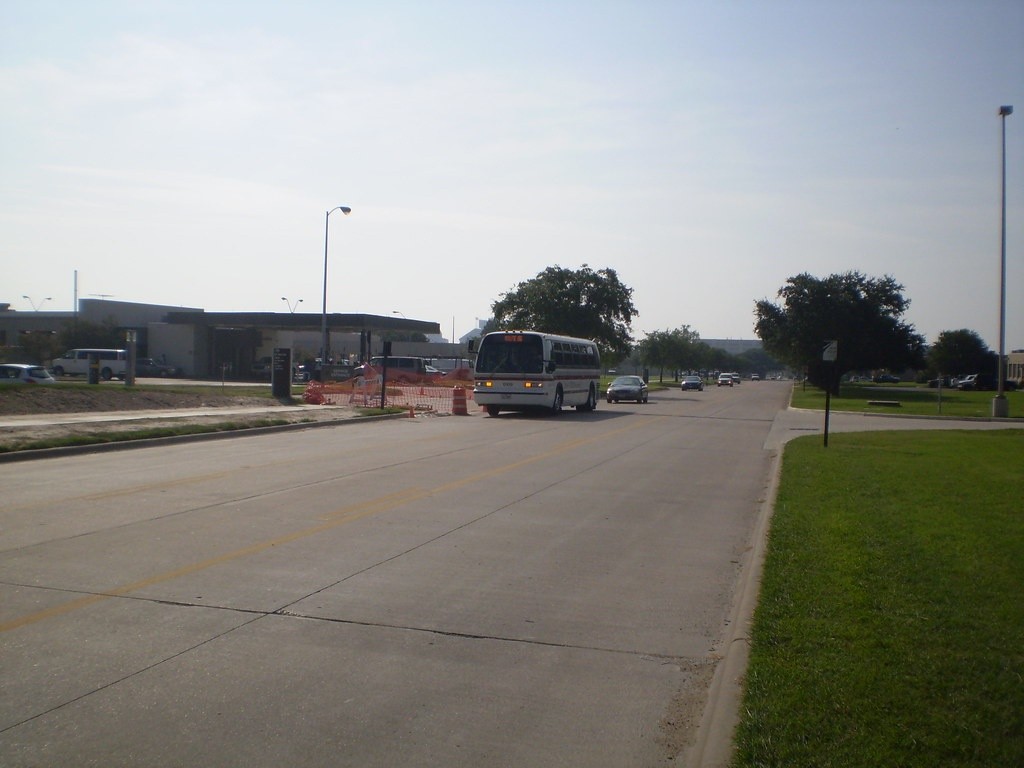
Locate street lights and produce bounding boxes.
[991,102,1015,417]
[321,205,352,385]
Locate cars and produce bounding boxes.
[0,363,55,384]
[957,373,978,390]
[606,375,650,404]
[873,372,900,384]
[752,374,759,381]
[718,372,740,387]
[251,355,298,382]
[771,376,775,381]
[680,375,704,392]
[135,357,177,379]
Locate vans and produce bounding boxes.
[52,348,126,381]
[353,356,425,385]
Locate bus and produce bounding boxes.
[472,329,602,416]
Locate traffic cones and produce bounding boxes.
[419,386,424,395]
[409,404,415,418]
